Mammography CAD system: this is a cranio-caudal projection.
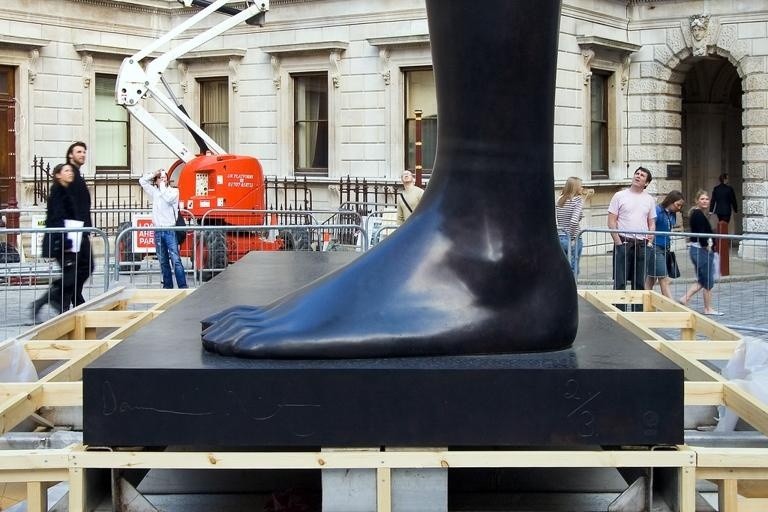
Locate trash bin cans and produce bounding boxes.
[115,222,141,271]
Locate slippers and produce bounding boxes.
[705,309,724,317]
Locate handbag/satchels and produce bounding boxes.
[63,219,85,254]
[667,251,680,277]
[175,211,186,244]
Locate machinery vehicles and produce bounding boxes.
[113,0,311,285]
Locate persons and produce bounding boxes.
[200,0,579,357]
[30,162,81,325]
[607,166,657,310]
[397,169,424,223]
[708,173,738,223]
[690,19,706,41]
[138,170,187,288]
[643,189,685,301]
[65,142,94,306]
[679,189,724,315]
[556,176,584,275]
